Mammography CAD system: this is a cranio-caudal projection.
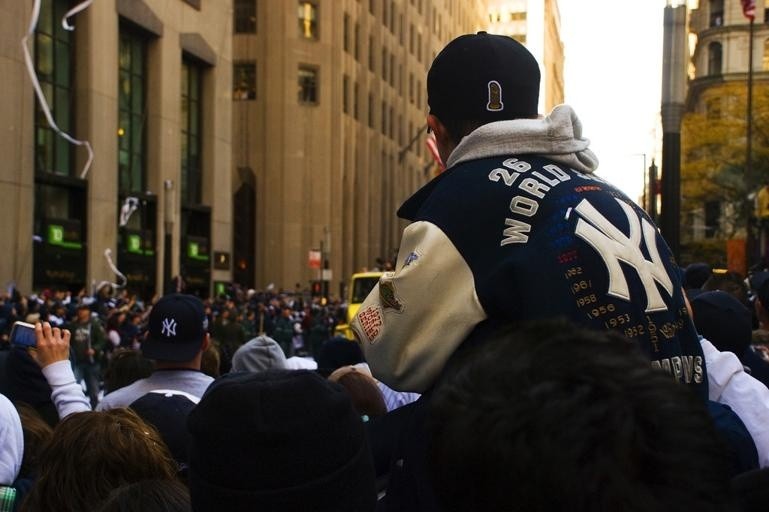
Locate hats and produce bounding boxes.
[685,288,754,354]
[197,371,365,493]
[127,388,202,469]
[425,30,539,118]
[143,293,212,361]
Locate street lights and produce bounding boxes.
[160,180,179,295]
[661,3,688,257]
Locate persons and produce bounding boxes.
[349,30,709,405]
[1,260,768,512]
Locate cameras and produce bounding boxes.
[9,321,38,349]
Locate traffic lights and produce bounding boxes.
[312,281,322,297]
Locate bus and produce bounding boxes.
[345,270,392,342]
